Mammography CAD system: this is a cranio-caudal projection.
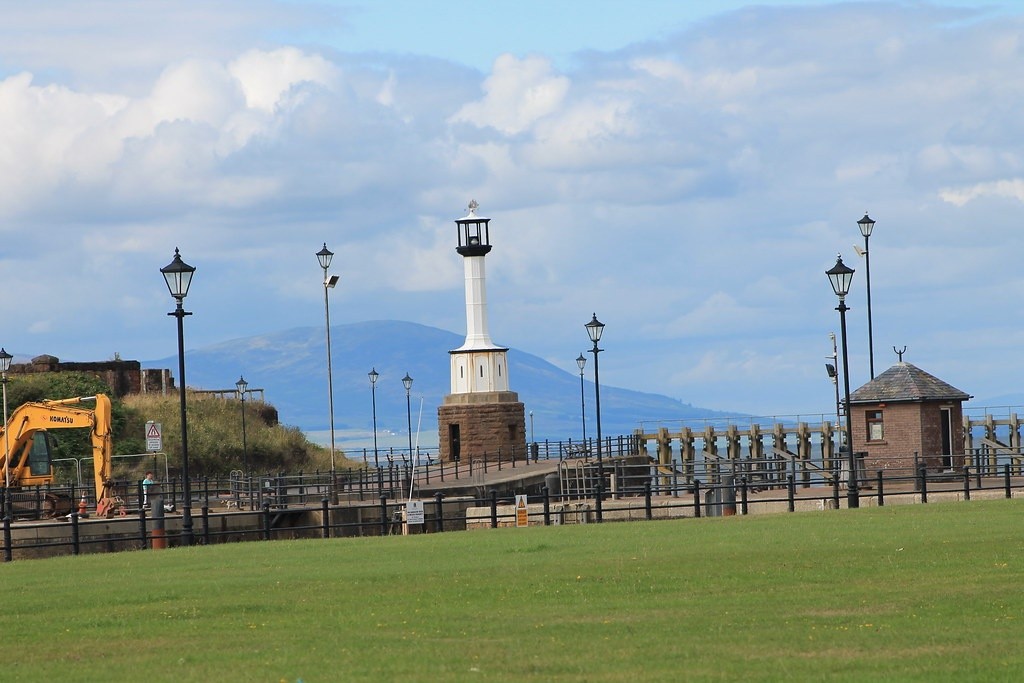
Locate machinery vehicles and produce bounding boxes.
[0,393,124,519]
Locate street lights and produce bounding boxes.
[367,366,381,472]
[234,375,260,510]
[582,312,610,501]
[824,253,867,509]
[401,371,417,477]
[157,246,200,545]
[575,351,590,463]
[854,211,876,382]
[0,348,13,562]
[528,411,535,445]
[824,332,843,449]
[316,239,346,506]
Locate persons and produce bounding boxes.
[142,471,152,508]
